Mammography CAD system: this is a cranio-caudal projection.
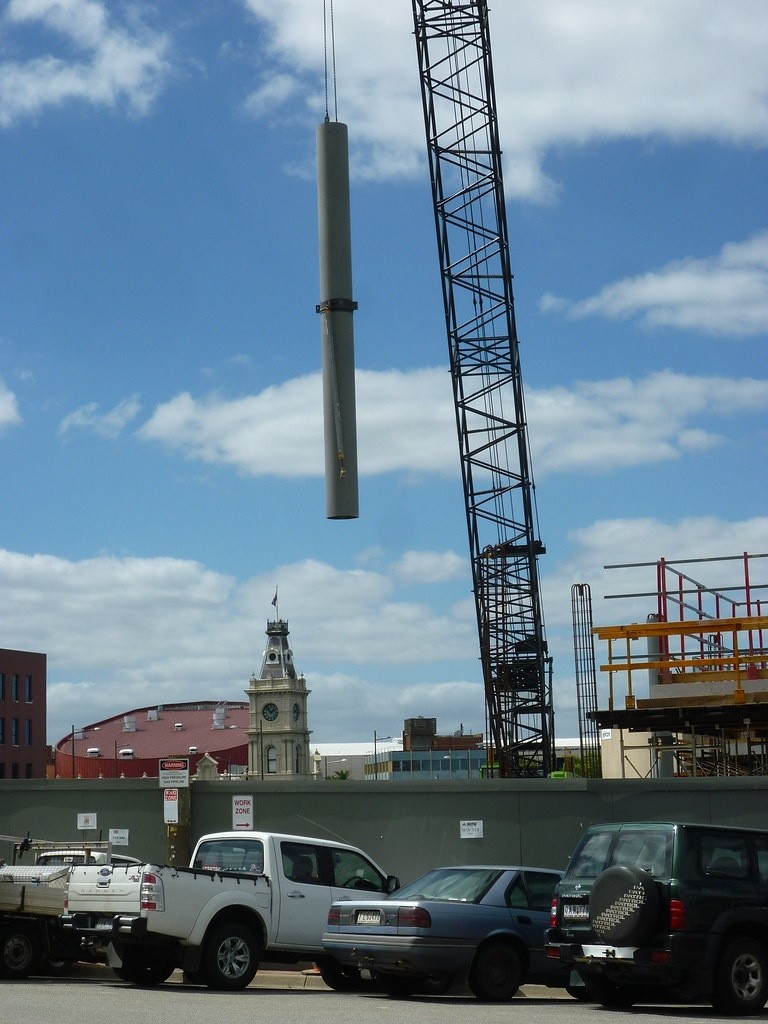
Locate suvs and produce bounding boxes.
[542,820,766,1016]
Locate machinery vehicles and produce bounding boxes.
[405,2,600,779]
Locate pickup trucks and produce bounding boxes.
[64,829,404,995]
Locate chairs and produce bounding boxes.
[292,856,318,885]
[531,893,553,910]
[707,856,740,878]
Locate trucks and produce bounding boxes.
[0,839,148,980]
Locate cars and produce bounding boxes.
[320,864,563,1003]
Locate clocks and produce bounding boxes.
[262,703,279,721]
[293,703,299,721]
